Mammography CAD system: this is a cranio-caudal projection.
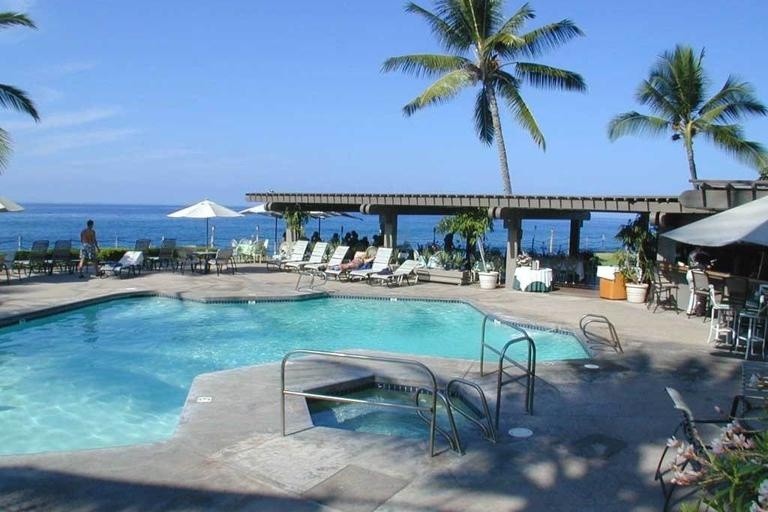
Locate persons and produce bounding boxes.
[344,232,350,243]
[76,219,104,279]
[311,231,323,242]
[338,244,377,271]
[689,246,712,264]
[282,232,286,241]
[539,240,549,257]
[351,231,359,240]
[686,253,712,317]
[329,233,340,242]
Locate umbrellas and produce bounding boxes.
[0,195,25,215]
[237,202,331,256]
[165,196,247,275]
[304,211,364,238]
[658,194,767,253]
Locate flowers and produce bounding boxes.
[664,419,766,512]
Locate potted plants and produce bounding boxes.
[434,208,498,289]
[616,213,652,303]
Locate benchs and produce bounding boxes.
[416,250,478,288]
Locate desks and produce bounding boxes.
[195,251,217,275]
[597,266,642,300]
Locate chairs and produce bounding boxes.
[655,385,759,512]
[96,238,153,279]
[529,246,591,290]
[208,245,237,278]
[646,258,682,316]
[229,236,269,264]
[324,251,369,283]
[43,238,72,280]
[370,259,420,287]
[13,239,49,281]
[266,239,310,274]
[284,241,328,274]
[1,240,19,285]
[348,247,393,285]
[688,269,767,361]
[145,238,177,273]
[303,245,349,280]
